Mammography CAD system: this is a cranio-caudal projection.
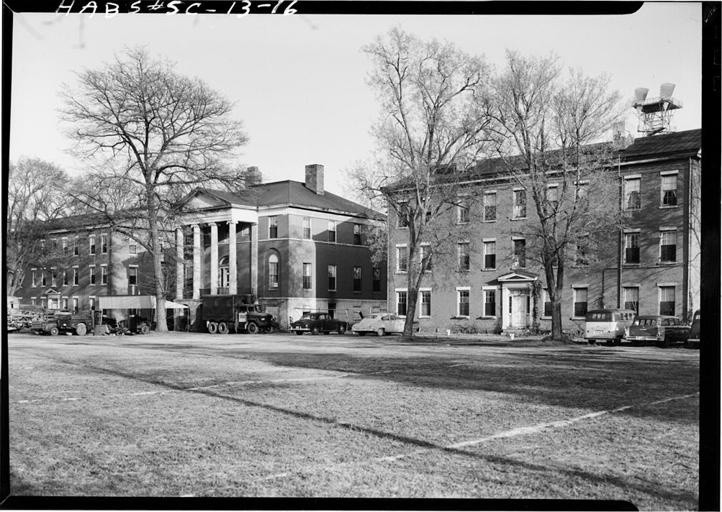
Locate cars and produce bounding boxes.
[626,315,692,342]
[289,313,347,335]
[351,312,405,336]
[30,310,117,335]
[686,309,700,348]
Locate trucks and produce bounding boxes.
[201,294,272,334]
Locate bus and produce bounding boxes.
[584,309,636,345]
